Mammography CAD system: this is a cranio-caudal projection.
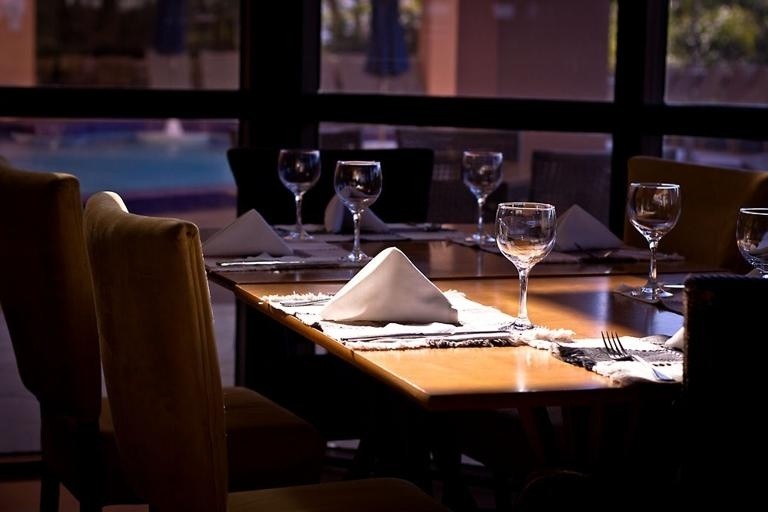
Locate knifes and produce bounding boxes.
[215,260,332,266]
[341,330,510,343]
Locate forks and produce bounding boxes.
[601,330,675,381]
[575,242,671,259]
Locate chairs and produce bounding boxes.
[315,125,614,227]
[507,277,767,509]
[621,155,768,277]
[1,155,313,512]
[228,148,434,494]
[529,150,611,229]
[397,128,518,225]
[81,191,441,512]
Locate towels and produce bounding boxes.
[202,208,294,256]
[324,186,391,233]
[553,204,625,251]
[318,247,465,326]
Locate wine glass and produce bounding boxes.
[627,181,683,300]
[462,150,504,242]
[334,160,382,263]
[734,207,768,276]
[493,201,558,333]
[277,148,322,241]
